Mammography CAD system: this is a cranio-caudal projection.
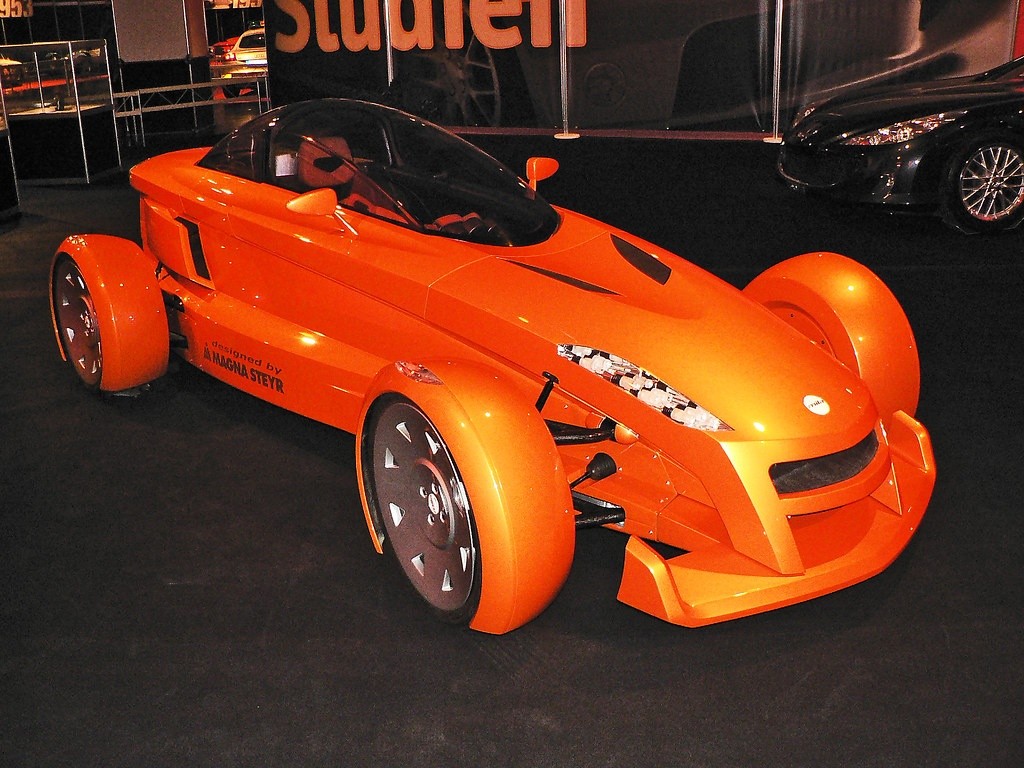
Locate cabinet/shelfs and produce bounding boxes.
[0,38,124,185]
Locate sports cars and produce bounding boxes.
[778,56,1024,239]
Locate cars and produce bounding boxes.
[220,27,269,99]
